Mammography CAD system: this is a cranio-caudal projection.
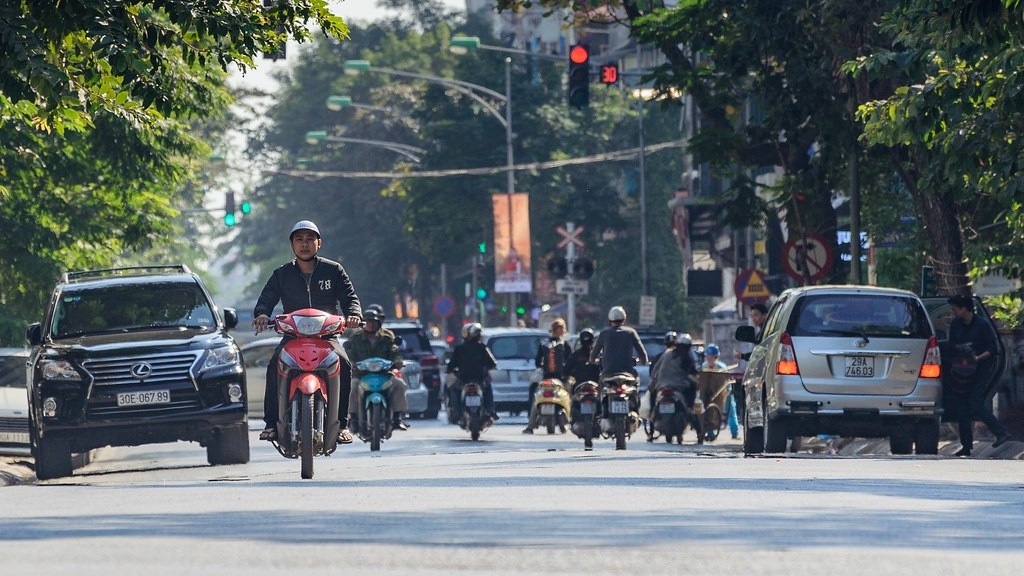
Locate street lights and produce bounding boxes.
[449,34,757,269]
[342,58,519,327]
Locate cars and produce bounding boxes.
[231,306,451,420]
[0,347,35,454]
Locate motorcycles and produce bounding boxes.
[342,335,407,453]
[252,307,368,480]
[442,356,498,441]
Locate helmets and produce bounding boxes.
[676,333,692,345]
[705,345,718,356]
[366,304,383,315]
[362,310,380,321]
[461,323,474,338]
[608,307,626,321]
[550,319,565,331]
[663,331,677,344]
[467,323,481,338]
[290,221,320,240]
[580,328,594,344]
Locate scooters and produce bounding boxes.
[533,364,575,435]
[585,353,654,450]
[649,369,701,443]
[563,370,606,449]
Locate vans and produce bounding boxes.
[734,284,1009,454]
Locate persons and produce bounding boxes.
[589,307,651,426]
[736,302,769,364]
[700,344,739,440]
[937,292,1011,458]
[340,300,411,431]
[445,323,499,426]
[564,327,603,391]
[523,319,574,435]
[250,220,364,443]
[648,331,705,443]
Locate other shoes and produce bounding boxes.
[991,431,1012,448]
[490,411,499,420]
[522,428,533,434]
[400,420,410,427]
[956,447,971,456]
[560,425,566,434]
[393,418,407,430]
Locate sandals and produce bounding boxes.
[337,428,353,443]
[260,427,278,440]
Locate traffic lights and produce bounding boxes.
[599,64,619,84]
[224,191,235,226]
[241,202,251,214]
[568,45,590,107]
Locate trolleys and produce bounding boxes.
[644,367,734,440]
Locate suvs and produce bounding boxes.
[26,264,250,481]
